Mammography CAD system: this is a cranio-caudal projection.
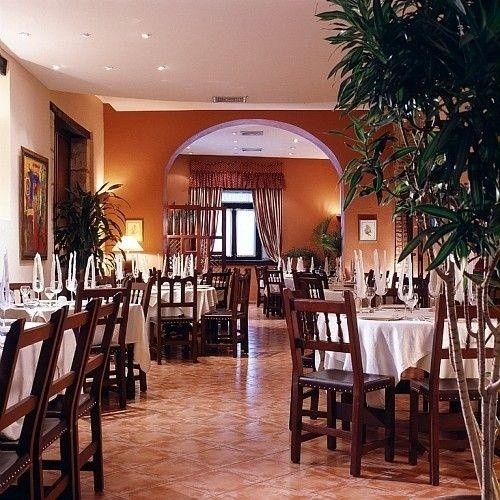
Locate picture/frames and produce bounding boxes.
[18,148,49,261]
[358,220,377,242]
[124,219,143,238]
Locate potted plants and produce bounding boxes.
[52,182,130,287]
[285,249,313,270]
[315,219,342,260]
[316,1,500,499]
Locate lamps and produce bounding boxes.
[113,236,143,253]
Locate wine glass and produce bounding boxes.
[375,284,388,312]
[366,287,375,307]
[21,286,30,300]
[133,271,138,282]
[398,284,413,320]
[168,272,173,279]
[405,293,419,320]
[44,288,54,304]
[50,281,63,307]
[32,280,44,301]
[0,291,10,331]
[66,281,77,304]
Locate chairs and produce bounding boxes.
[392,273,422,306]
[156,270,198,365]
[292,270,329,371]
[0,309,65,500]
[201,268,251,358]
[283,288,394,477]
[265,267,286,319]
[45,292,123,500]
[255,265,268,307]
[0,300,77,500]
[72,280,131,411]
[200,268,231,309]
[95,276,154,399]
[409,294,500,485]
